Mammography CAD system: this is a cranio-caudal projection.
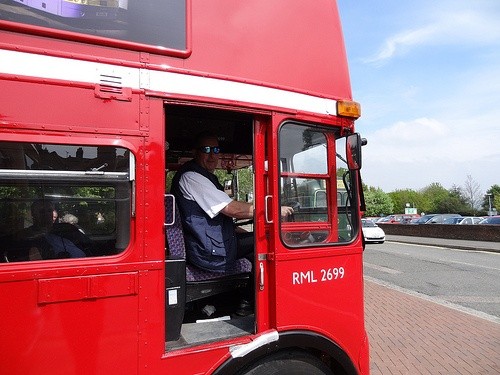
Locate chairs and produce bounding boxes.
[162,194,252,312]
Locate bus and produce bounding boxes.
[11,0,119,20]
[280,177,351,242]
[0,0,369,375]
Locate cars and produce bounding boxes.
[361,219,385,244]
[365,213,500,225]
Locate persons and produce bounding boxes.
[168,135,294,317]
[0,200,85,263]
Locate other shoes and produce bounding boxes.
[235,297,253,314]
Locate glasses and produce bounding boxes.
[197,146,220,154]
